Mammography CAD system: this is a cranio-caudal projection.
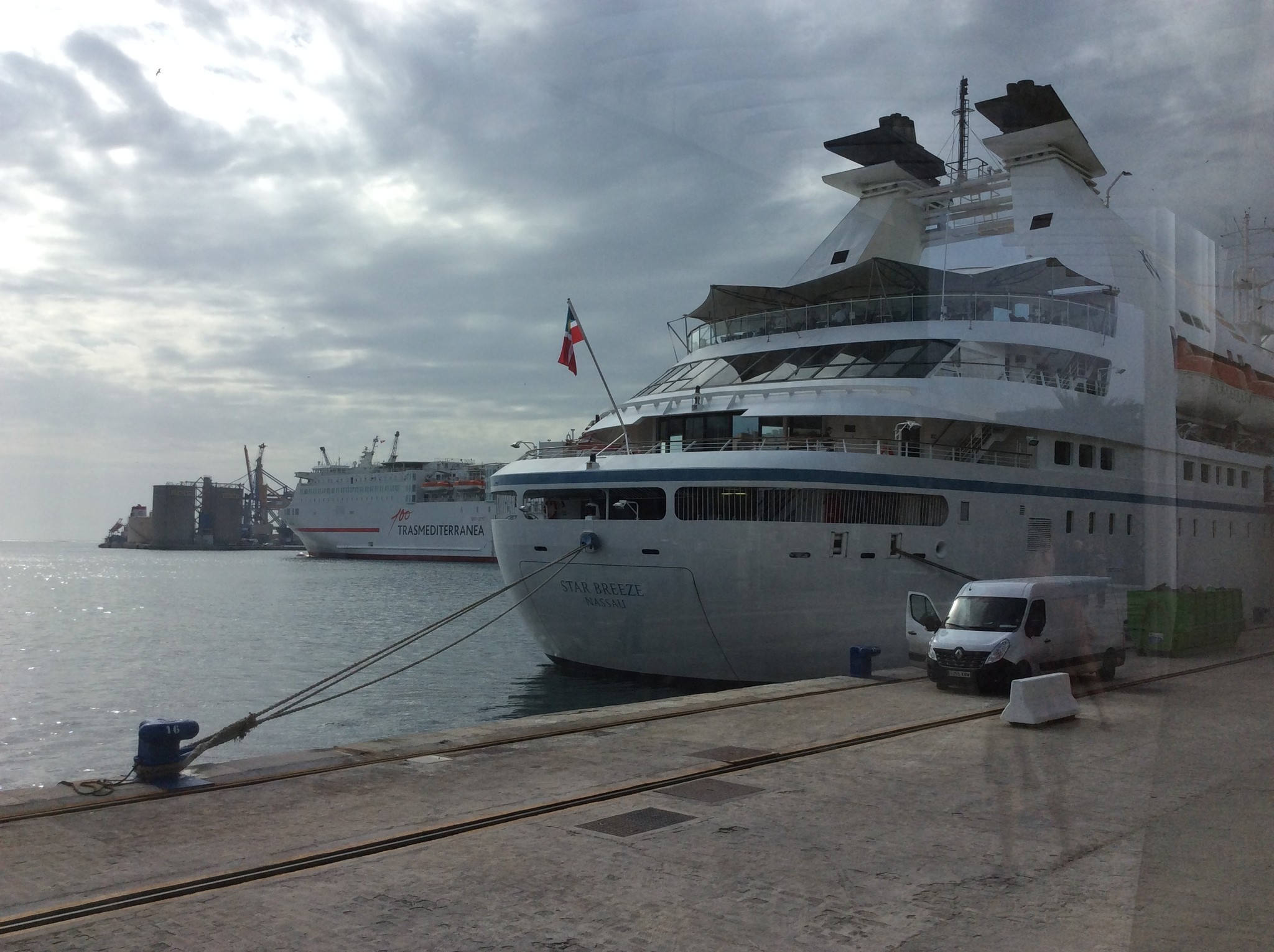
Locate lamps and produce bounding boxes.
[508,440,536,455]
[1097,365,1126,380]
[519,501,550,519]
[612,500,640,520]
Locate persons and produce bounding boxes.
[1027,305,1099,333]
[766,316,776,335]
[831,303,850,327]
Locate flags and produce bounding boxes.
[558,305,585,376]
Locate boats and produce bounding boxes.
[1175,337,1251,424]
[269,431,504,565]
[1234,364,1274,436]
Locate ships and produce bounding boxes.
[486,81,1273,681]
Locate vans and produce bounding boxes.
[908,574,1131,694]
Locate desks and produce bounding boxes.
[877,314,891,322]
[815,321,831,328]
[773,326,785,333]
